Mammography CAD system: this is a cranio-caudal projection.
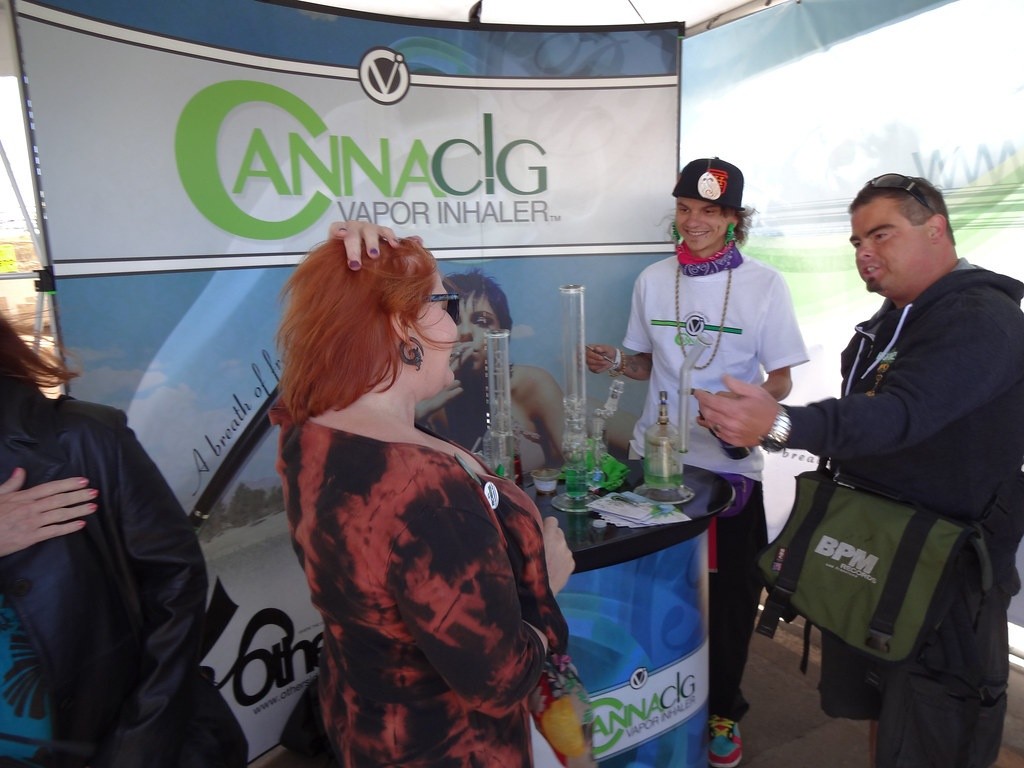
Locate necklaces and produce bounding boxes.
[676,266,731,370]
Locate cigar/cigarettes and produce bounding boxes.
[452,352,460,356]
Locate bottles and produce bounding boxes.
[645,391,684,489]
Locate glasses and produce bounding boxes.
[423,293,460,325]
[866,173,935,215]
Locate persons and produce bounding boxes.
[693,174,1024,768]
[583,158,808,768]
[268,222,574,768]
[413,272,585,483]
[0,318,250,768]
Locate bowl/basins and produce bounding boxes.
[531,468,561,493]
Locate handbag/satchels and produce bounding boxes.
[755,467,980,669]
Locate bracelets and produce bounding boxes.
[609,349,621,370]
[607,349,626,377]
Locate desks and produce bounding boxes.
[519,458,736,768]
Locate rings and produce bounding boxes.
[715,425,720,432]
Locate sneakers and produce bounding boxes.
[707,712,742,768]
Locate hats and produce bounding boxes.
[671,157,745,211]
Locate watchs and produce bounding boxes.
[761,408,793,452]
